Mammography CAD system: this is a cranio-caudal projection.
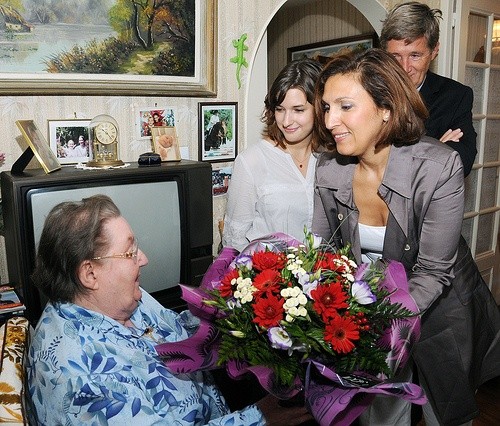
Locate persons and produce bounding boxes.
[310,47,500,426]
[140,112,169,136]
[56,126,89,157]
[221,60,336,252]
[26,195,270,426]
[206,110,229,144]
[380,1,478,179]
[27,124,59,172]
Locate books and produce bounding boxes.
[0,282,26,316]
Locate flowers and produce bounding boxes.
[200,224,420,393]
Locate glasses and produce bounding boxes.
[92,242,139,264]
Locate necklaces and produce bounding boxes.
[290,152,312,169]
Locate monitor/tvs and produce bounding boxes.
[0,158,213,322]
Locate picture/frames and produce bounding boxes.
[287,32,379,65]
[0,0,218,98]
[151,126,180,162]
[198,102,238,163]
[10,120,62,176]
[47,119,92,165]
